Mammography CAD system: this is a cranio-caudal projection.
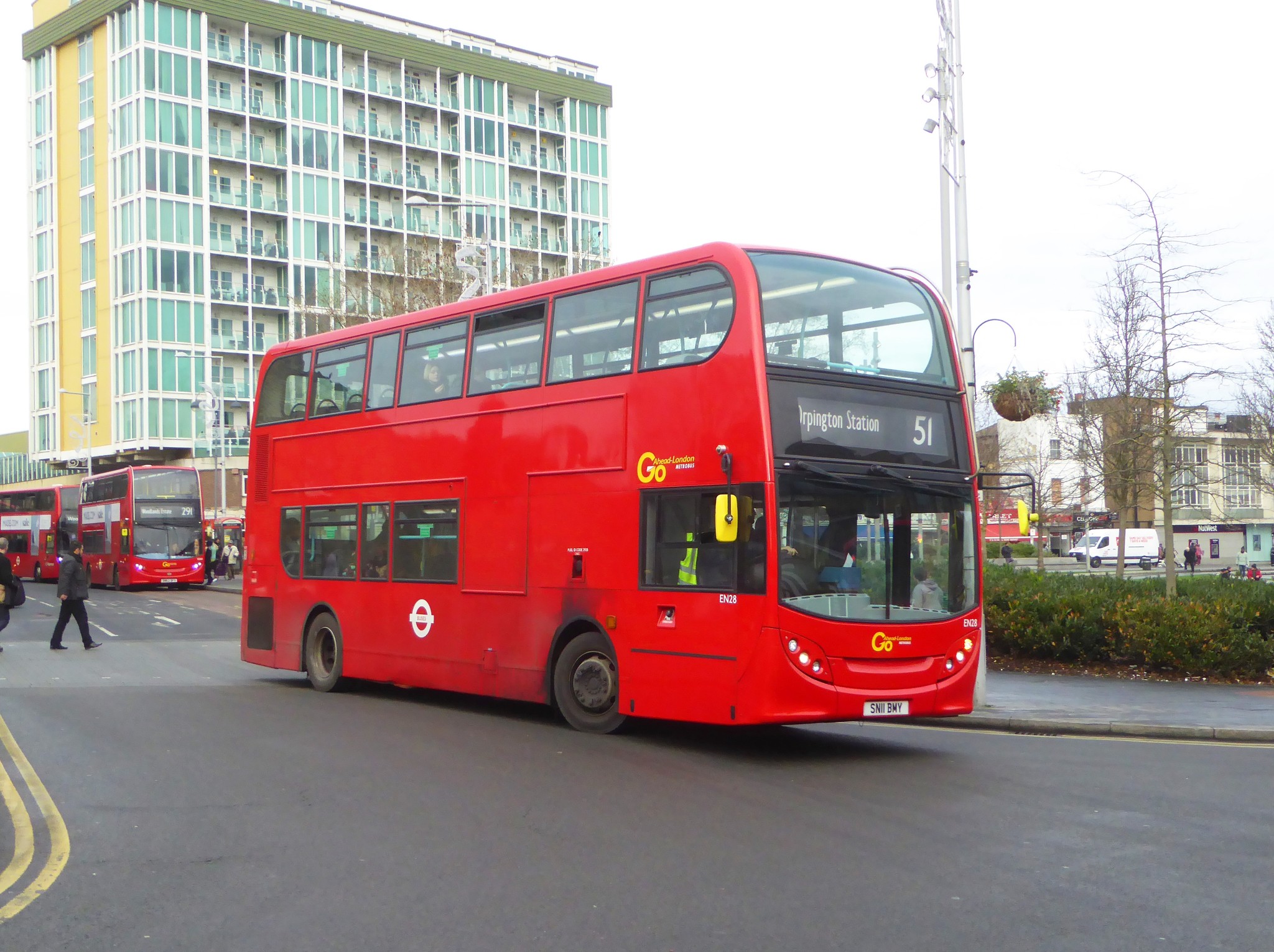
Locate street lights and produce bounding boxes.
[59,389,94,477]
[175,351,227,515]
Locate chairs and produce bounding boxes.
[288,354,707,418]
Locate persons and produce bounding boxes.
[205,538,239,585]
[107,487,111,499]
[1270,544,1274,567]
[1154,543,1178,567]
[912,567,944,609]
[282,517,322,577]
[170,526,186,556]
[739,506,799,565]
[1221,566,1231,581]
[372,557,388,578]
[1184,543,1204,572]
[1247,564,1262,581]
[0,538,18,652]
[1001,542,1013,564]
[50,540,103,650]
[411,359,489,401]
[1235,546,1249,579]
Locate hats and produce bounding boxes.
[206,540,213,547]
[228,540,234,543]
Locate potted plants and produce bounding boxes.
[976,365,1064,422]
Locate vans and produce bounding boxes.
[1069,528,1160,568]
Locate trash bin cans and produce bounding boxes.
[1142,557,1151,571]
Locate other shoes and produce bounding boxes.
[50,643,69,650]
[212,578,218,582]
[228,577,232,580]
[204,582,211,585]
[85,642,103,650]
[0,646,3,652]
[232,575,235,579]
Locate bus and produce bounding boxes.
[238,242,1039,735]
[75,463,214,592]
[1,485,80,583]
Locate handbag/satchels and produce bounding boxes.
[216,562,227,576]
[0,584,5,603]
[222,555,229,564]
